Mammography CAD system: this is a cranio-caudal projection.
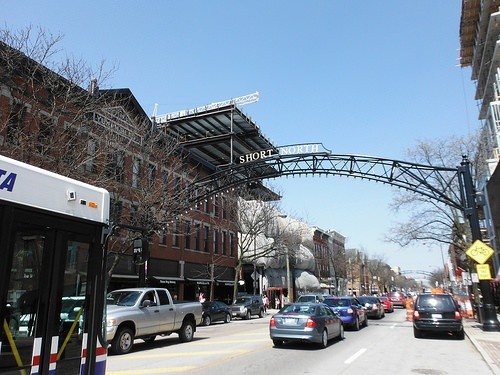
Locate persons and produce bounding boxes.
[262,295,269,314]
[199,292,205,304]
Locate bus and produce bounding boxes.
[0,151,112,375]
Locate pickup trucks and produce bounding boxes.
[102,286,204,355]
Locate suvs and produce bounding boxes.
[229,294,266,320]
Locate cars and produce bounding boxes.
[356,296,386,320]
[377,295,394,313]
[17,295,86,337]
[321,296,369,331]
[269,301,346,348]
[385,290,436,309]
[295,289,333,312]
[199,300,233,326]
[410,292,465,340]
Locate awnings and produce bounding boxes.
[79,271,245,286]
